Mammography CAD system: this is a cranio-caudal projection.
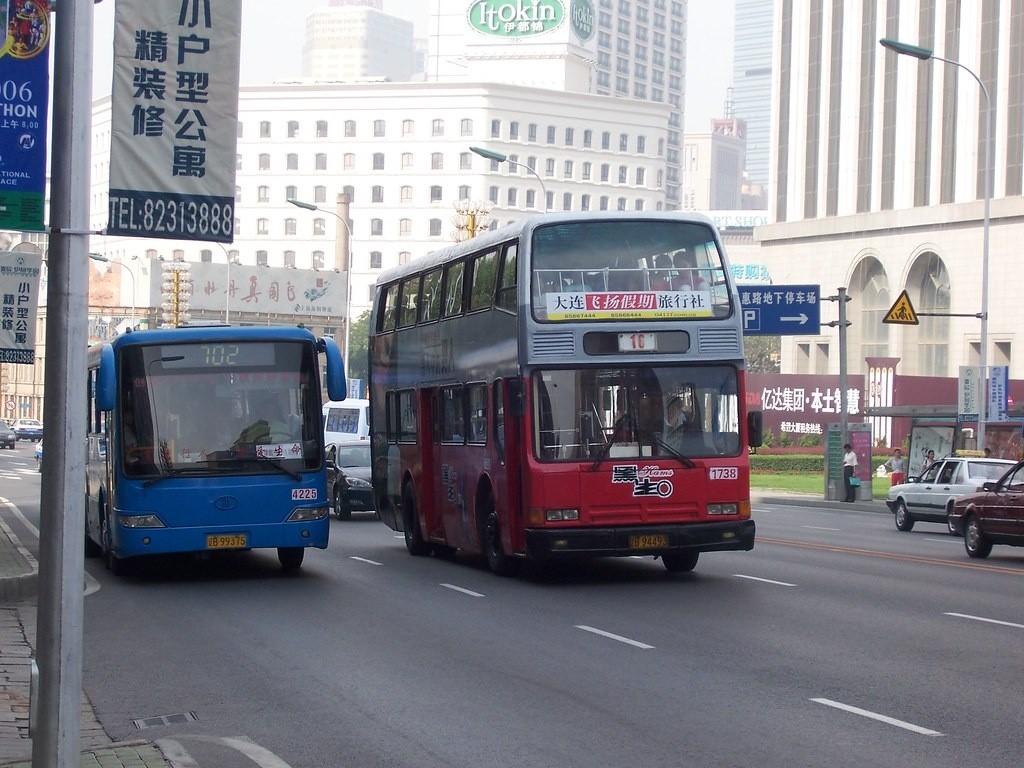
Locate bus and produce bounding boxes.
[368,210,765,580]
[84,321,347,577]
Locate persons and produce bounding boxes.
[842,444,858,502]
[123,406,138,463]
[242,404,291,443]
[918,449,937,480]
[651,395,688,433]
[984,448,992,458]
[884,449,906,485]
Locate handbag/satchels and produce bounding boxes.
[849,477,860,487]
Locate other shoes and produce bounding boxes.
[840,499,847,502]
[847,499,854,502]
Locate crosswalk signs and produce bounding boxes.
[882,289,919,325]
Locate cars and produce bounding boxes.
[323,440,376,522]
[0,421,16,449]
[2,418,43,443]
[34,437,44,473]
[950,458,1024,559]
[886,449,1024,536]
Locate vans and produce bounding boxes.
[320,397,371,443]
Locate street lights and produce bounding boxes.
[879,38,993,451]
[469,144,548,215]
[89,253,136,330]
[287,197,350,400]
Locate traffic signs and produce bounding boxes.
[737,284,820,335]
